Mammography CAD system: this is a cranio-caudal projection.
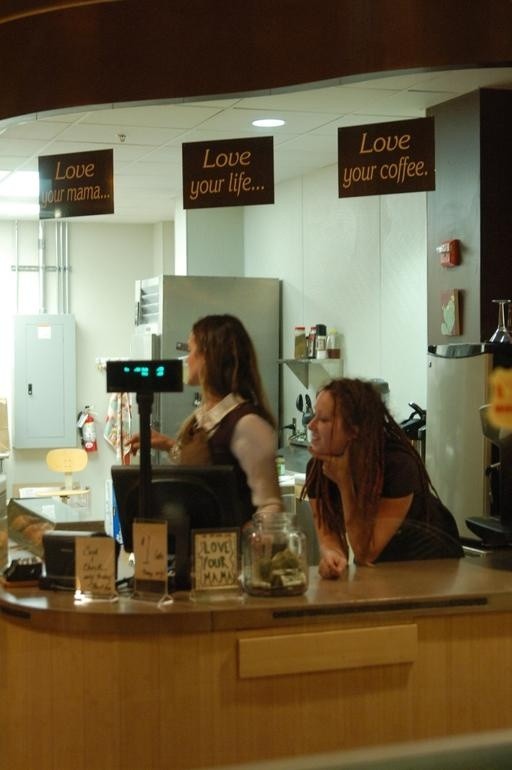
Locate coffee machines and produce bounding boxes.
[398,401,425,469]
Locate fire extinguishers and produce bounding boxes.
[78,406,98,452]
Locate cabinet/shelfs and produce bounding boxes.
[8,311,80,451]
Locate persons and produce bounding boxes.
[305,377,467,580]
[120,311,283,530]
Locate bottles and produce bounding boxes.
[308,326,317,358]
[293,327,308,358]
[242,511,310,596]
[316,324,327,358]
[327,329,341,359]
[276,455,286,475]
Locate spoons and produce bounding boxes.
[296,395,305,424]
[302,395,315,418]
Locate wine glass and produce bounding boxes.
[489,300,510,344]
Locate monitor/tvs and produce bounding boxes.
[109,465,254,596]
